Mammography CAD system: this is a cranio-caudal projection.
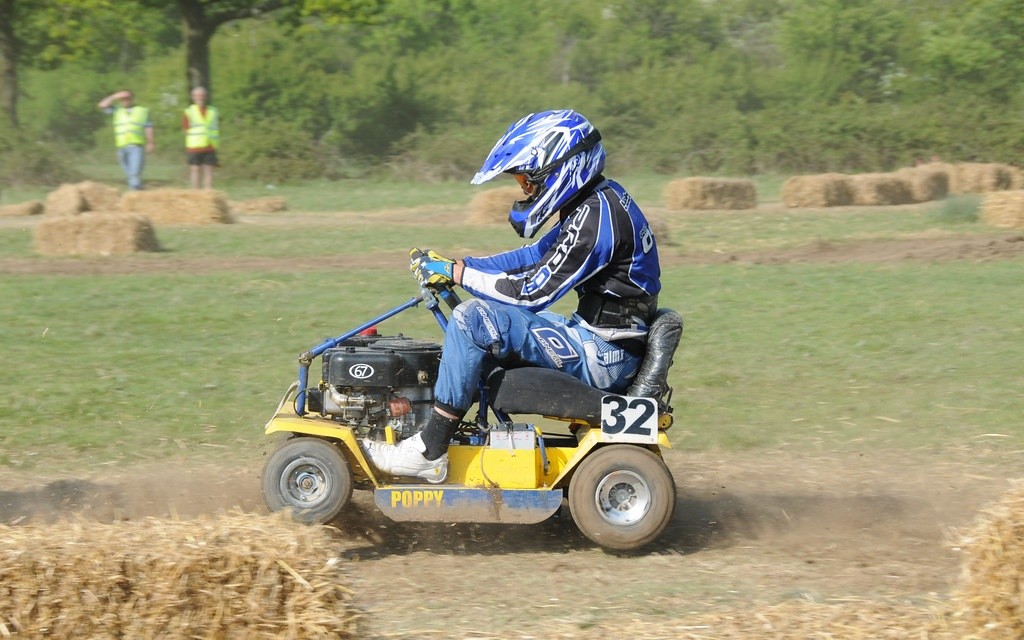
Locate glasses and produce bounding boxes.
[513,172,540,198]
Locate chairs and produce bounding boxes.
[489,308,683,421]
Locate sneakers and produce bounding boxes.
[362,430,449,484]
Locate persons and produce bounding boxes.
[98,89,154,189]
[182,86,221,190]
[361,110,661,484]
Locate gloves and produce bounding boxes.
[410,249,456,289]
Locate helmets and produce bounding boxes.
[470,109,606,238]
